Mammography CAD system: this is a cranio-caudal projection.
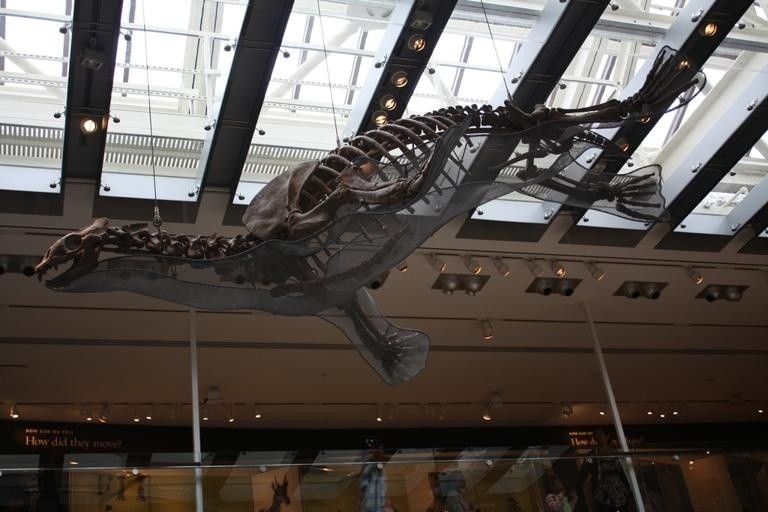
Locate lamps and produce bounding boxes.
[392,253,706,286]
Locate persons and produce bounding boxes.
[540,472,578,512]
[443,470,471,512]
